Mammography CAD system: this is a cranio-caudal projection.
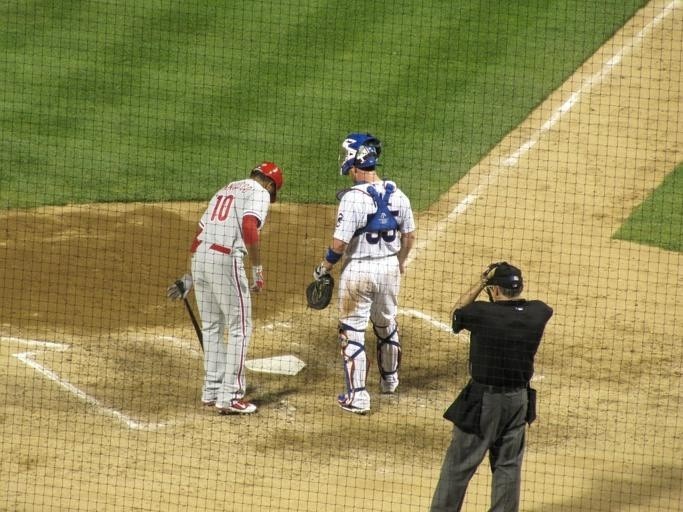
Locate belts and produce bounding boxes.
[482,385,527,392]
[196,240,231,254]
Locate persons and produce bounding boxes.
[310,133,417,417]
[164,161,282,417]
[425,260,555,512]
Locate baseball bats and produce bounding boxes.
[174,280,203,348]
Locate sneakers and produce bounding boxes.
[338,394,370,413]
[380,381,398,393]
[204,399,258,415]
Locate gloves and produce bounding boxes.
[251,266,263,292]
[167,274,193,302]
[313,264,330,282]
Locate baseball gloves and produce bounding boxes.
[306,274,334,309]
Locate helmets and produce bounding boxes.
[480,262,521,302]
[251,163,282,203]
[338,134,381,175]
